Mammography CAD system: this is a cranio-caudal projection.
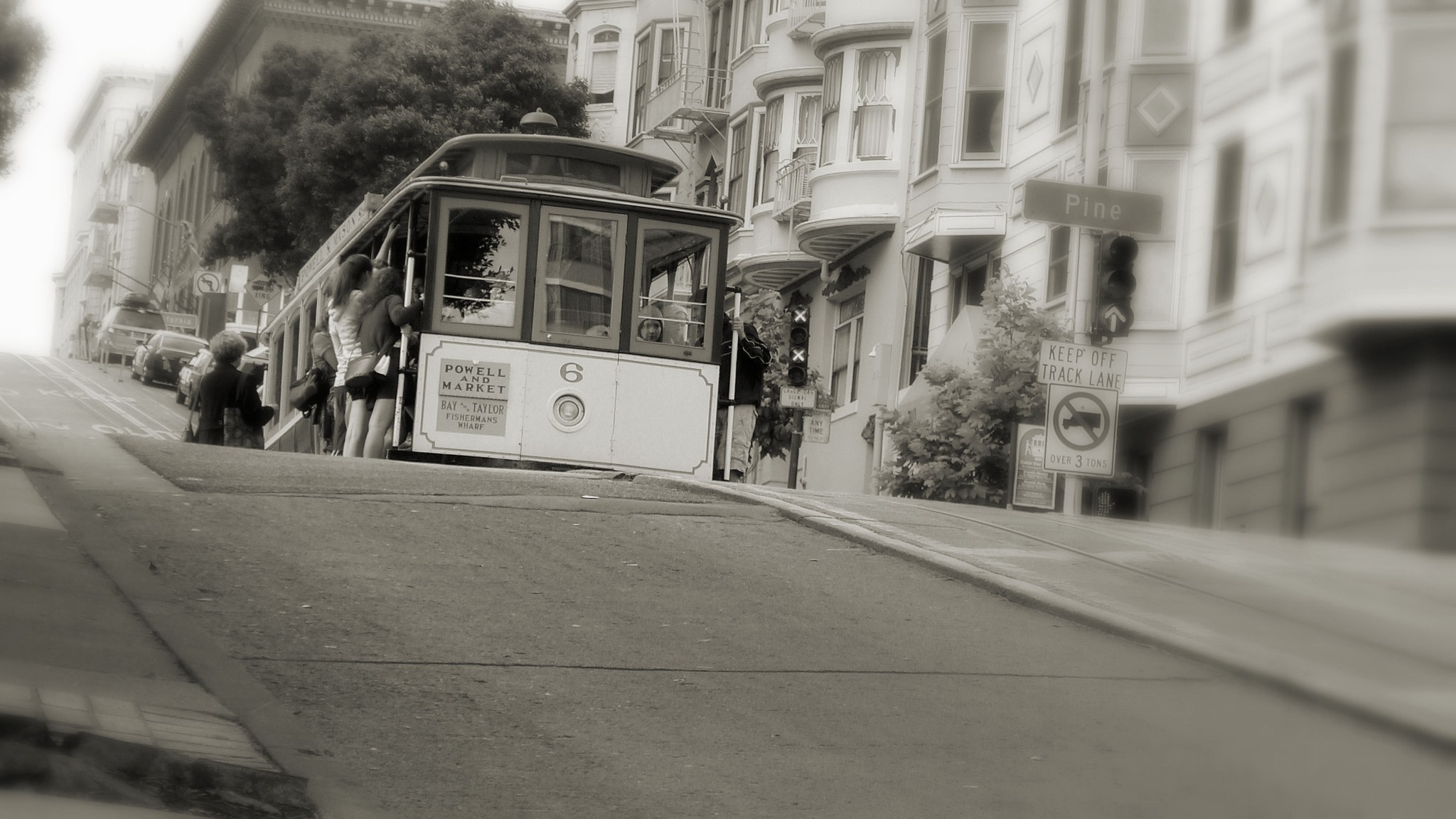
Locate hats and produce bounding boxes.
[584,325,610,337]
[637,305,664,335]
[662,304,688,319]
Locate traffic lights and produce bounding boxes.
[1092,232,1139,337]
[788,305,811,386]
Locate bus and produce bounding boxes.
[260,108,745,483]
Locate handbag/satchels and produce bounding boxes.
[288,371,323,411]
[344,351,380,388]
[224,407,265,450]
[180,424,197,443]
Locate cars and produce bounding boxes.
[132,329,265,412]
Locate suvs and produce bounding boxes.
[92,306,166,363]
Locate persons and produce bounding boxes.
[339,265,427,461]
[661,305,690,348]
[323,220,401,460]
[451,287,488,316]
[178,327,280,457]
[410,277,426,307]
[689,287,772,483]
[368,258,389,279]
[299,295,341,458]
[638,305,664,347]
